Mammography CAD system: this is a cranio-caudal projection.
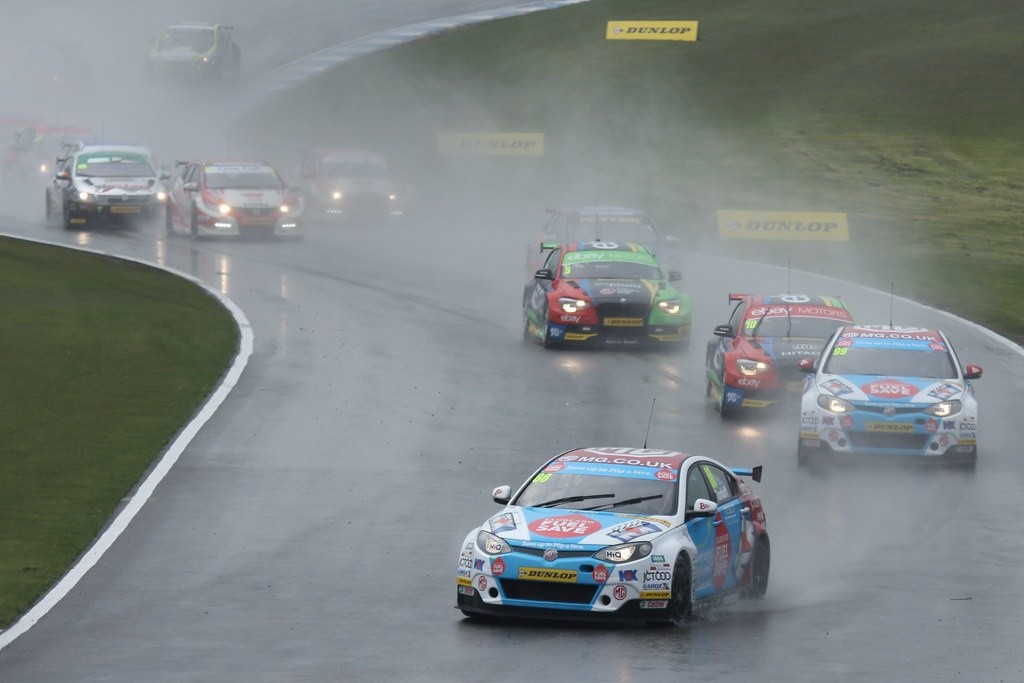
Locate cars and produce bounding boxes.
[43,142,173,230]
[549,210,683,299]
[300,149,404,226]
[455,445,771,632]
[521,241,691,350]
[797,323,979,467]
[164,160,302,244]
[704,292,856,418]
[147,21,242,86]
